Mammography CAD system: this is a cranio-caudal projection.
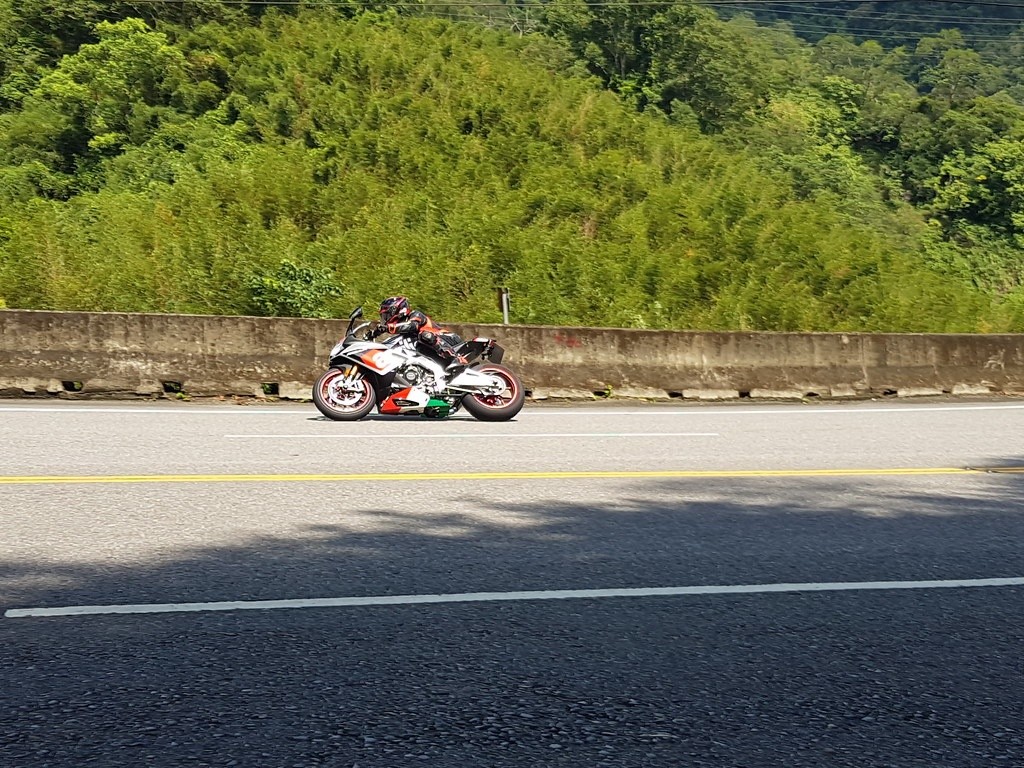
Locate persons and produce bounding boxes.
[367,297,468,372]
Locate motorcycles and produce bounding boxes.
[313,306,525,421]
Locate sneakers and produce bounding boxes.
[444,353,468,373]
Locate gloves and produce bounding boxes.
[374,324,388,336]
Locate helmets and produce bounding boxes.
[379,296,410,324]
